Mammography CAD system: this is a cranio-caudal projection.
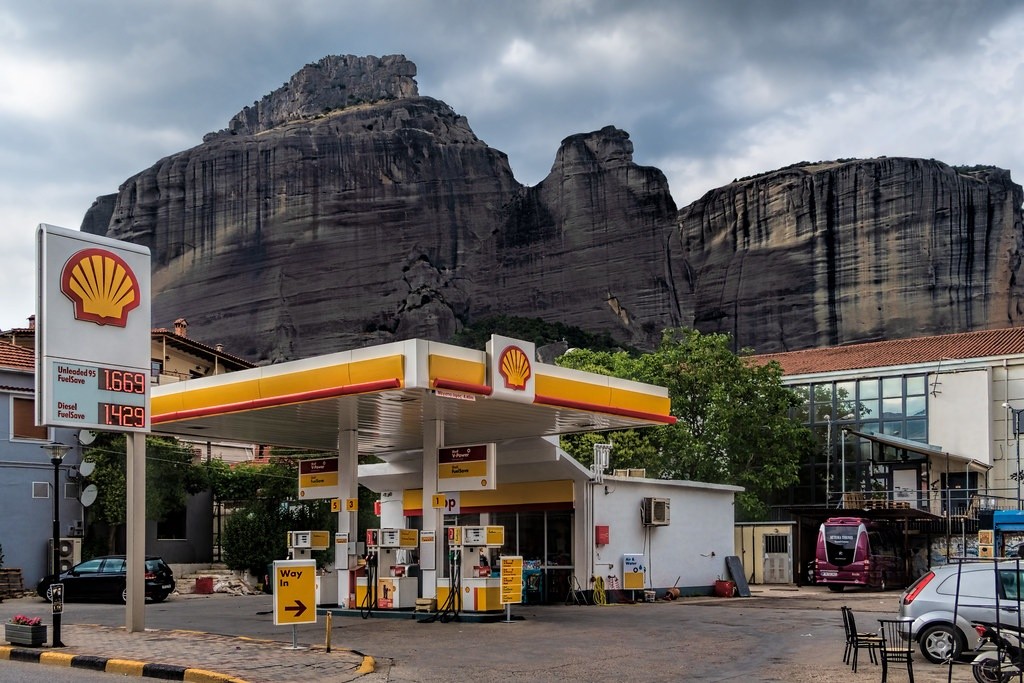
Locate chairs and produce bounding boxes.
[840,605,915,683]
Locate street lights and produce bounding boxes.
[41,441,74,649]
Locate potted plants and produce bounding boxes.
[715,573,736,599]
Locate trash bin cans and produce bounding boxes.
[194,576,214,594]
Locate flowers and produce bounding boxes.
[9,613,42,625]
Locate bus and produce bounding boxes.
[814,516,910,591]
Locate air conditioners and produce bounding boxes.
[642,497,671,526]
[47,537,82,575]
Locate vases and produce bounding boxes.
[5,622,48,645]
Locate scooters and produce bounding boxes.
[969,607,1024,683]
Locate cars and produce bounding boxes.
[37,555,175,606]
[895,558,1024,665]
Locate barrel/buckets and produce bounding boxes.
[196,578,213,594]
[644,591,656,602]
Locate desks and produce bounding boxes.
[539,565,573,569]
